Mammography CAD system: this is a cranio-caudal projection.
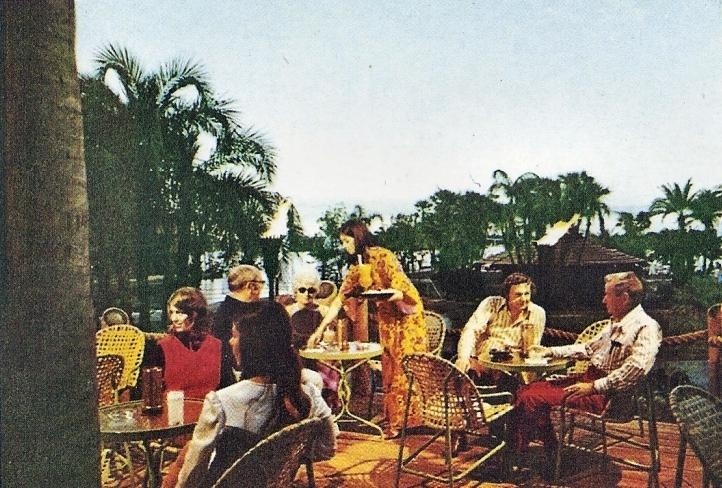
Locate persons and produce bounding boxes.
[441,271,549,458]
[476,270,664,484]
[303,219,432,439]
[91,263,343,418]
[159,296,339,488]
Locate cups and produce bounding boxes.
[167,389,185,425]
[141,365,163,413]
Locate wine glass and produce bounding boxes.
[358,263,375,293]
[324,331,336,346]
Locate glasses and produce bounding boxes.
[245,280,267,285]
[297,286,317,294]
